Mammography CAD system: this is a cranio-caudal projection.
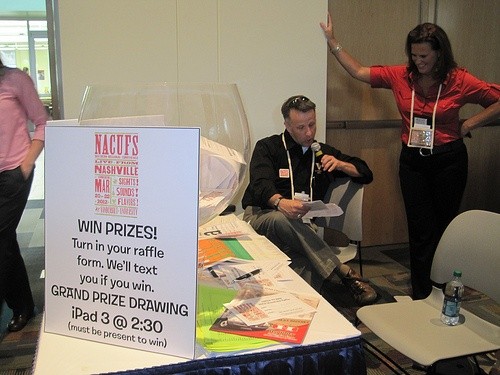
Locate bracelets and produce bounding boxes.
[273,196,282,209]
[331,41,343,53]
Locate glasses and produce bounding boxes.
[288,97,308,107]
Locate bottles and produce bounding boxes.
[440,270,464,326]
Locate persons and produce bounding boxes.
[243,94,378,304]
[320,11,499,300]
[0,55,51,333]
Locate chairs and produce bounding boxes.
[314,177,364,276]
[355,210,500,375]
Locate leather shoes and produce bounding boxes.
[6,313,27,331]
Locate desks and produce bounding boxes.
[30,214,368,375]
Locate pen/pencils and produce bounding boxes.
[236,268,261,281]
[209,267,218,278]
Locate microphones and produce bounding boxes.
[311,142,334,182]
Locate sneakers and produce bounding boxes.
[339,265,377,308]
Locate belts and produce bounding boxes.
[402,138,464,157]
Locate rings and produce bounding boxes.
[297,213,301,218]
